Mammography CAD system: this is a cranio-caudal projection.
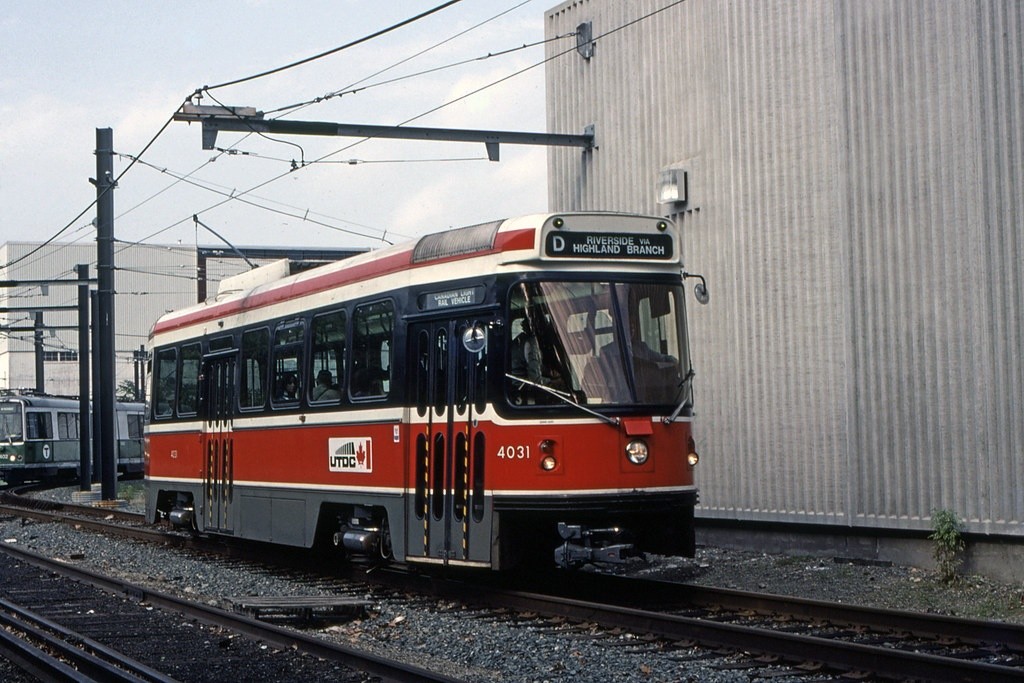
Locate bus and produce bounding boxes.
[140,211,708,577]
[0,386,146,487]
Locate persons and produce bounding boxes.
[312,369,340,401]
[512,309,598,404]
[275,375,297,401]
[600,315,678,365]
[349,349,390,397]
